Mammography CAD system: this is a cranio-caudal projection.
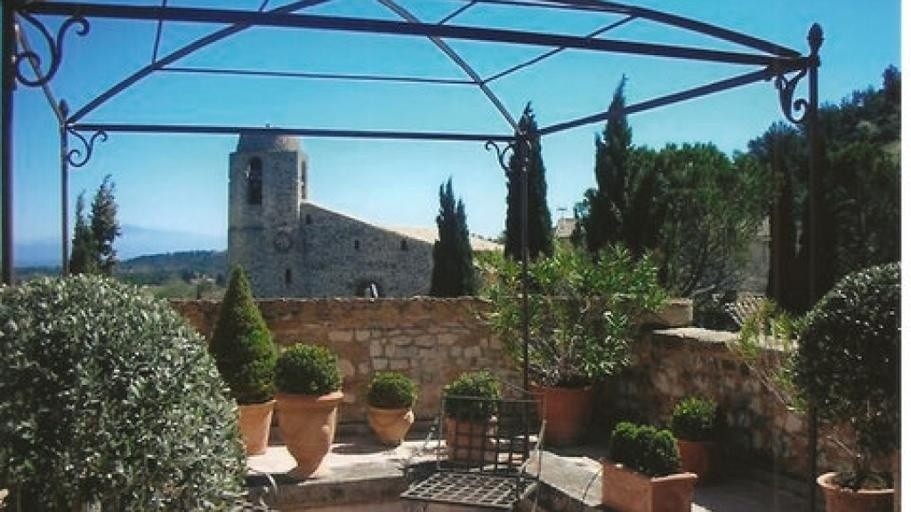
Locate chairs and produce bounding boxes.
[396,394,548,512]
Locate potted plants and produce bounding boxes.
[365,372,414,444]
[668,397,719,485]
[469,242,667,445]
[786,256,902,512]
[272,344,344,455]
[443,369,500,463]
[600,421,699,512]
[214,267,281,454]
